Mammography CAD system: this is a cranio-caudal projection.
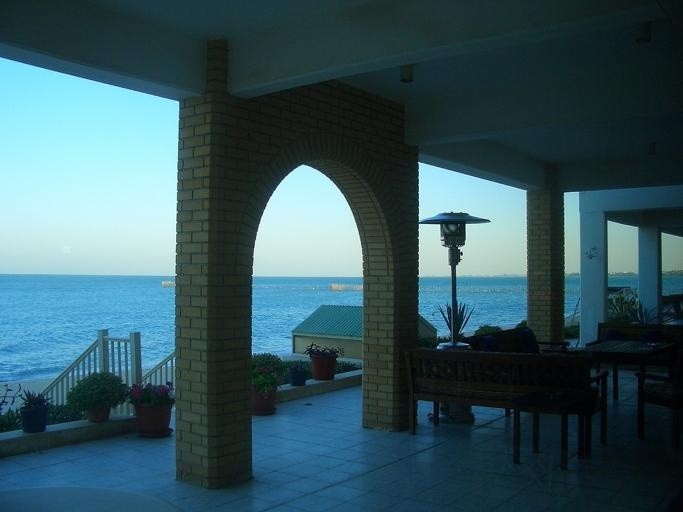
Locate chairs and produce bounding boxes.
[632,341,682,447]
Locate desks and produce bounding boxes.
[542,343,612,388]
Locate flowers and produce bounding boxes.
[126,380,175,406]
[303,342,345,359]
[249,360,281,398]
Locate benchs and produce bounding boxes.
[586,319,682,400]
[462,326,540,418]
[415,345,611,470]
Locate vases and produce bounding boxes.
[285,366,308,386]
[132,400,174,437]
[309,354,337,380]
[249,381,277,415]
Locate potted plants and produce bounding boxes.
[64,368,129,423]
[19,388,53,433]
